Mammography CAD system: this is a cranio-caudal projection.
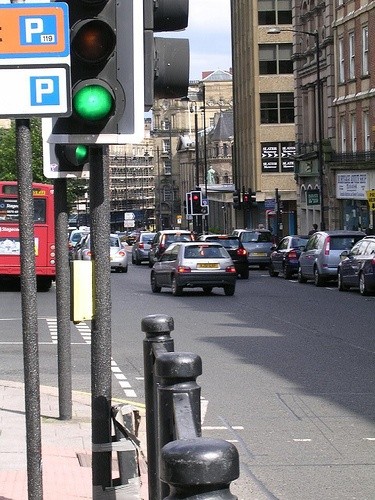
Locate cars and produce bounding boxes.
[75,234,127,273]
[150,241,236,295]
[268,235,309,279]
[147,230,195,268]
[336,235,375,295]
[131,233,157,264]
[196,235,248,278]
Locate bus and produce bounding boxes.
[0,180,56,291]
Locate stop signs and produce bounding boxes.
[0,2,72,119]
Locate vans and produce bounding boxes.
[231,229,276,269]
[296,230,367,286]
[69,229,89,256]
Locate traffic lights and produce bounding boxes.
[243,195,250,210]
[185,193,190,215]
[143,1,189,111]
[190,191,209,216]
[42,0,145,145]
[41,143,91,179]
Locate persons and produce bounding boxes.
[308,223,319,235]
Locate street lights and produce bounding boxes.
[266,28,324,231]
